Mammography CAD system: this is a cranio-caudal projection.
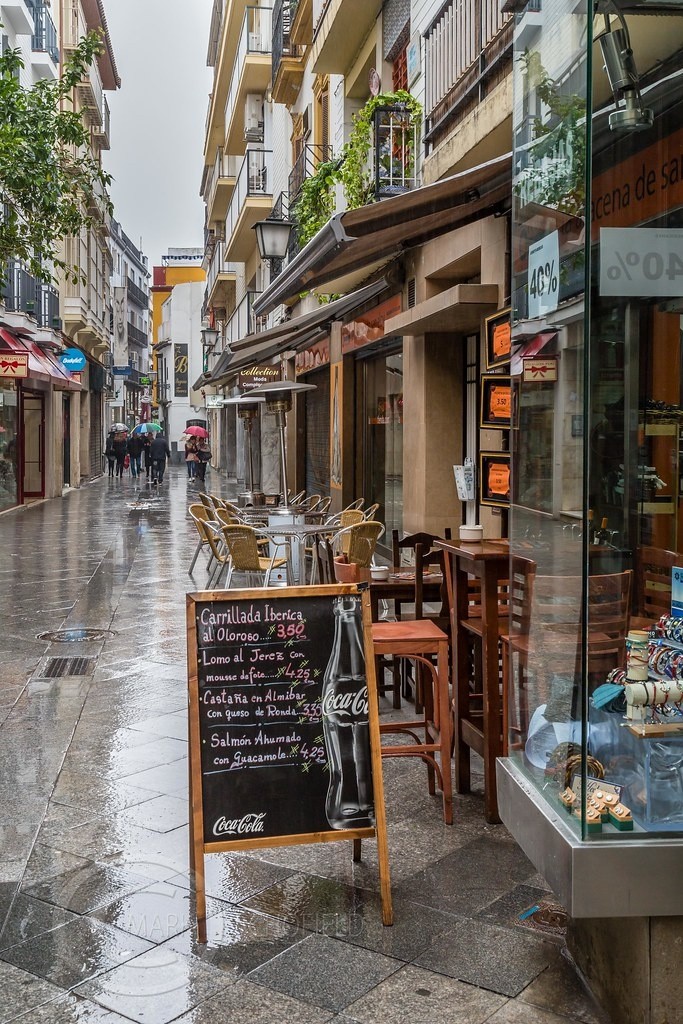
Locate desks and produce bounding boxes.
[358,563,476,700]
[238,504,310,513]
[247,510,326,524]
[432,534,631,824]
[256,524,343,586]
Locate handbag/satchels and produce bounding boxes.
[202,452,212,461]
[194,455,199,464]
[123,455,130,469]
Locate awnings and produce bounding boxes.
[192,267,403,392]
[251,100,618,316]
[1,328,83,391]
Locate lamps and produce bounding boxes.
[590,2,655,133]
[250,189,299,273]
[147,362,157,384]
[200,325,223,356]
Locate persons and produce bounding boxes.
[196,437,211,481]
[149,432,170,486]
[185,436,198,482]
[106,432,154,478]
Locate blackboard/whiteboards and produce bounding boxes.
[185,581,385,853]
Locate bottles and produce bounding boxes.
[588,509,593,546]
[593,518,608,546]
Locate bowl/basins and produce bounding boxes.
[459,524,483,542]
[369,565,389,580]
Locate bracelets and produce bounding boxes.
[606,613,683,724]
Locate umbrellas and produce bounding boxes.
[131,423,163,433]
[183,426,209,438]
[108,423,130,433]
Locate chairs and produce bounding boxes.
[189,486,634,825]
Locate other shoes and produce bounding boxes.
[192,478,195,481]
[188,478,192,481]
[200,476,205,481]
[133,474,136,478]
[145,474,149,477]
[137,473,140,479]
[159,482,163,486]
[154,478,158,485]
